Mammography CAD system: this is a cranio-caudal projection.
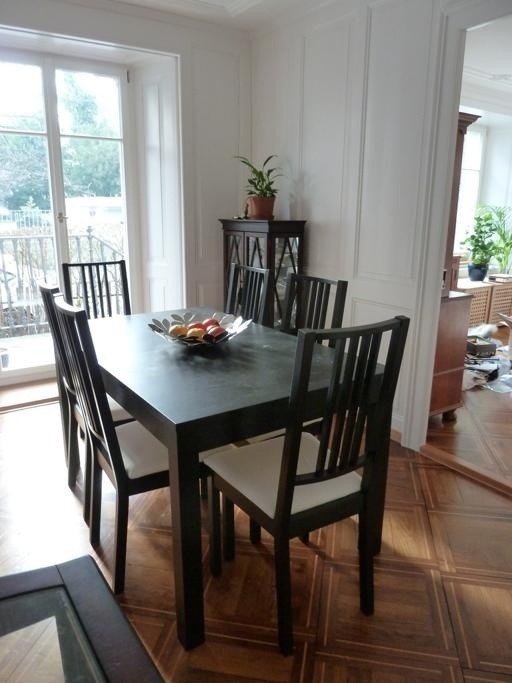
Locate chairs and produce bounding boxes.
[62,261,131,319]
[203,314,410,659]
[40,284,136,521]
[51,293,222,595]
[226,263,274,329]
[279,273,349,348]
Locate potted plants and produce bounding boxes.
[232,154,285,221]
[459,208,504,281]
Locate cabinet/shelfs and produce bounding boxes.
[430,290,472,423]
[217,217,307,334]
[427,110,481,297]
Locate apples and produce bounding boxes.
[168,319,225,339]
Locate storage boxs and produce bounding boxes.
[491,322,510,345]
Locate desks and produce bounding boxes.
[0,553,165,682]
